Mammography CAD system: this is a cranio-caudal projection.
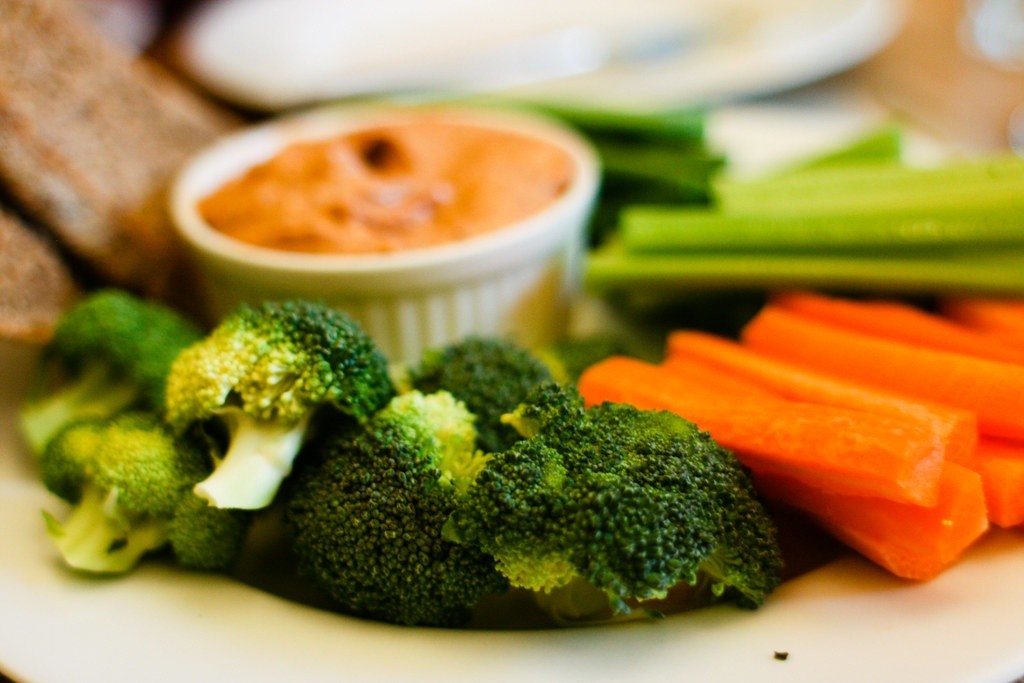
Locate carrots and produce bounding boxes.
[577,288,1024,579]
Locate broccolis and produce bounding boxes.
[19,293,782,623]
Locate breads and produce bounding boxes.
[0,0,233,343]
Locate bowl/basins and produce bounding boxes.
[167,100,601,360]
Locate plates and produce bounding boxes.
[0,1,1024,683]
